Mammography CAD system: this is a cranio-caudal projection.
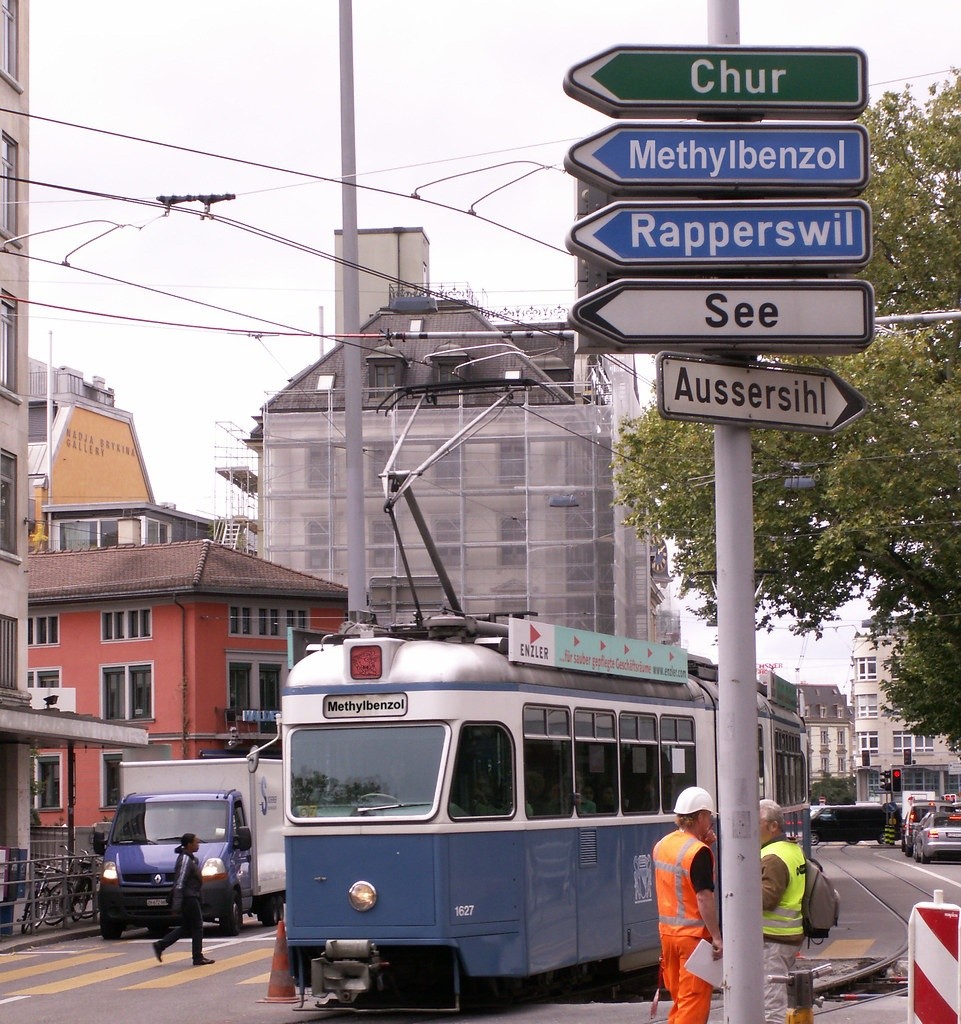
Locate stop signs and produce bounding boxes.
[819,796,826,804]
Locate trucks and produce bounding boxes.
[92,757,287,940]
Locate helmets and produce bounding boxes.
[673,786,718,815]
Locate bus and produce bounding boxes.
[247,379,812,1012]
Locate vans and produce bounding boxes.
[811,807,902,846]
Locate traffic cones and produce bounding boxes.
[256,921,308,1004]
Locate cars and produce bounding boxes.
[902,802,961,864]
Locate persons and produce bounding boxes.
[153,834,216,966]
[451,739,672,816]
[652,786,723,1024]
[758,799,807,1024]
[820,809,835,819]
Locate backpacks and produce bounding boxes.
[798,844,841,938]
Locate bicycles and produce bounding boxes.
[21,845,103,934]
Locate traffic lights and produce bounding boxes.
[885,770,892,791]
[944,794,951,801]
[880,771,888,789]
[950,794,956,803]
[891,768,902,793]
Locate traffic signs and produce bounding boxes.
[565,122,871,196]
[565,200,875,275]
[656,351,868,436]
[563,44,870,121]
[567,278,875,355]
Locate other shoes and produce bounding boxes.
[152,942,163,962]
[192,956,215,965]
